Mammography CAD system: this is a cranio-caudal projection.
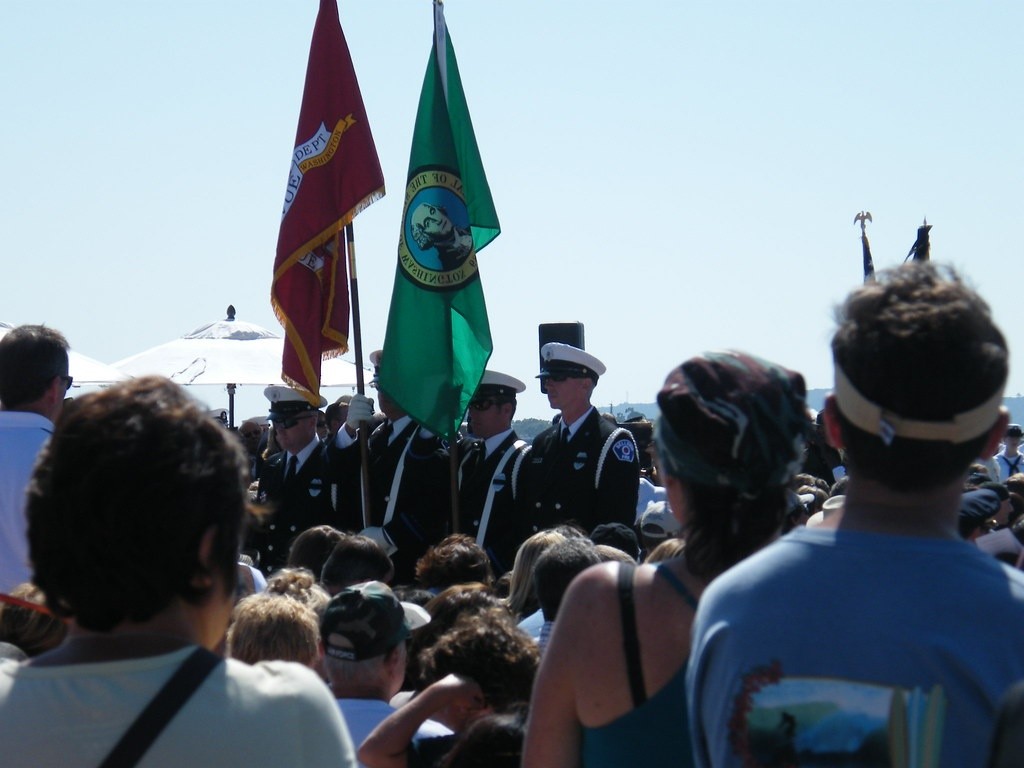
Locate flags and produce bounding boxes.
[376,0,502,440]
[270,0,386,408]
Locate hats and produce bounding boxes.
[264,386,327,421]
[1003,424,1024,437]
[640,501,676,538]
[474,369,526,401]
[535,342,607,386]
[321,580,432,661]
[210,408,229,426]
[326,395,353,425]
[657,352,805,500]
[959,489,1000,537]
[369,349,383,383]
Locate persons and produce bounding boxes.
[0,257,1024,768]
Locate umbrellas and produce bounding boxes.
[109,305,372,428]
[0,322,133,388]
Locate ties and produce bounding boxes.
[476,442,486,467]
[378,424,393,450]
[558,426,570,463]
[282,455,298,487]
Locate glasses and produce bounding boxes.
[540,377,566,382]
[318,421,326,428]
[470,399,506,410]
[273,415,312,428]
[62,377,73,389]
[245,430,260,438]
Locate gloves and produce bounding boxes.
[347,395,374,430]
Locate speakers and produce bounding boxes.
[539,323,584,394]
